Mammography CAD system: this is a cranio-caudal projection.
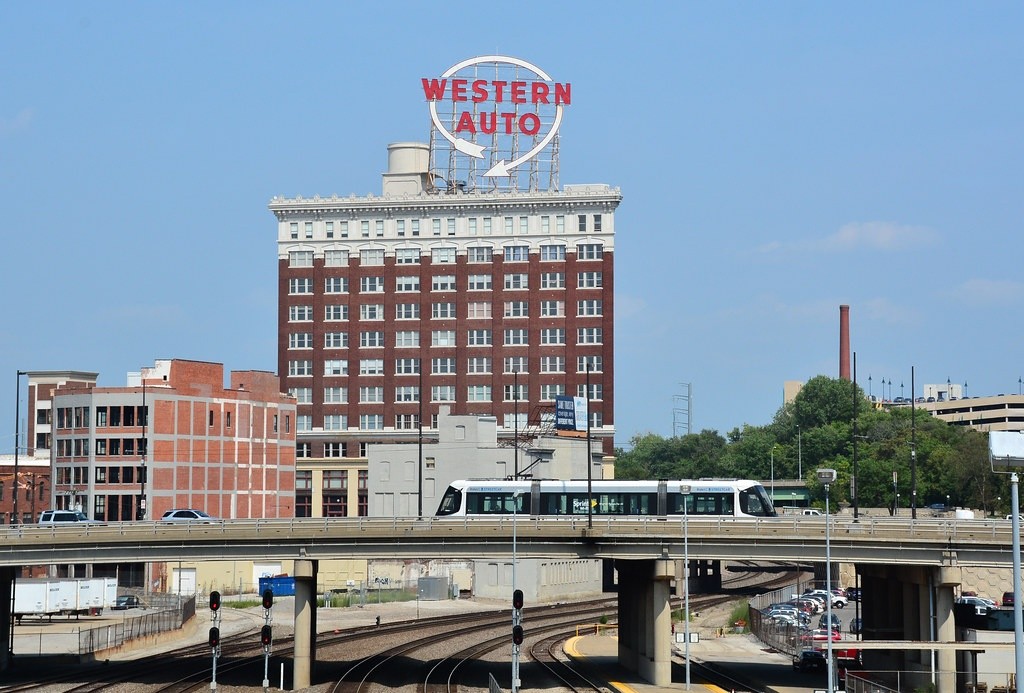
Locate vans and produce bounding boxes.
[803,509,822,517]
[1006,514,1024,521]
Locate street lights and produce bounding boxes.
[1018,377,1022,395]
[929,387,932,397]
[881,377,885,400]
[679,484,692,690]
[11,370,27,529]
[888,378,891,400]
[815,468,838,693]
[796,425,801,481]
[868,373,872,396]
[964,379,968,397]
[771,446,776,507]
[900,381,903,403]
[947,376,951,399]
[512,489,525,693]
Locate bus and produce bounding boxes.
[431,456,781,523]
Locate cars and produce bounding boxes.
[867,393,1021,404]
[759,585,872,682]
[111,594,140,610]
[955,591,1014,616]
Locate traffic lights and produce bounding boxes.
[210,591,221,611]
[209,627,219,647]
[261,625,271,645]
[262,588,272,609]
[513,590,524,645]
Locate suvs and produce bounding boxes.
[36,508,223,528]
